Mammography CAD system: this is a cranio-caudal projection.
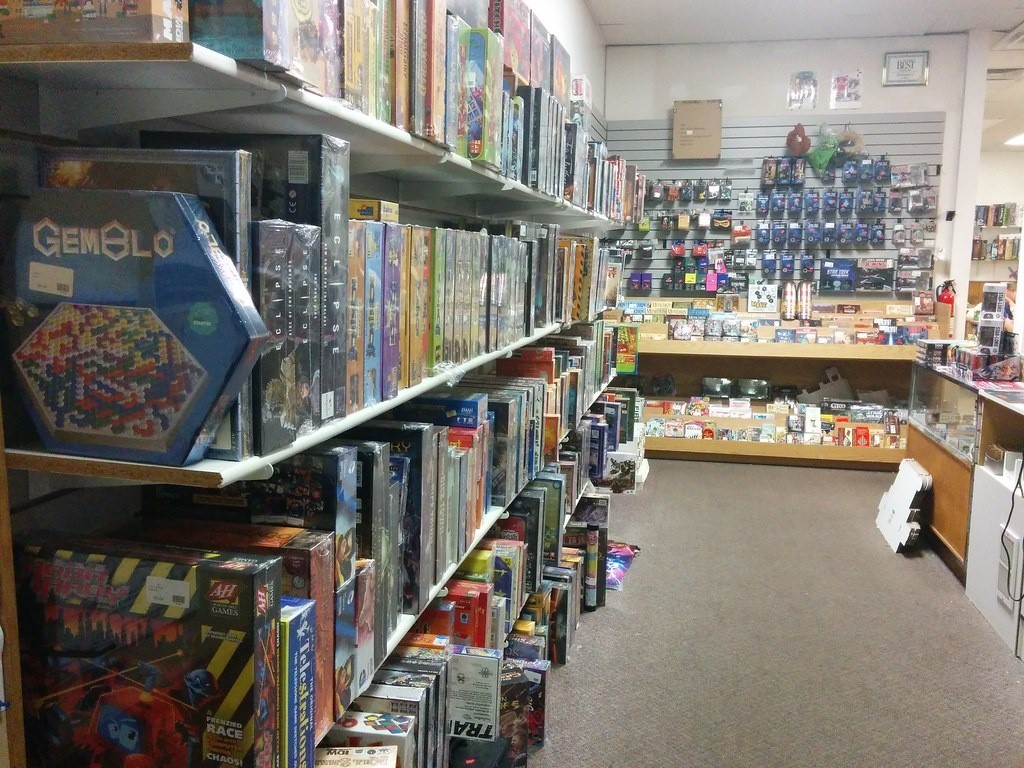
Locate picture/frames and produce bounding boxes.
[881,51,930,85]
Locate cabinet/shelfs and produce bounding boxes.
[0,41,624,767]
[965,390,1024,664]
[622,297,954,472]
[902,357,1022,587]
